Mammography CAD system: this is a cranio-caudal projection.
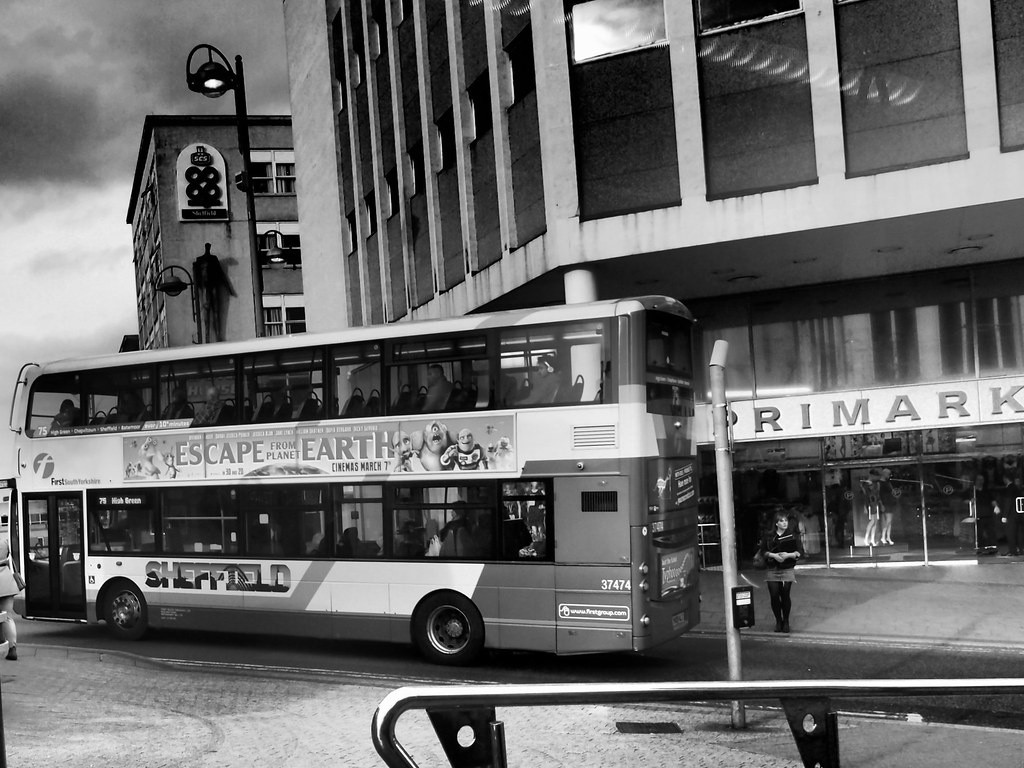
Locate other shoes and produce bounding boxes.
[774,619,790,633]
[1002,551,1024,556]
[5,646,17,660]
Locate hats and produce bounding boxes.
[397,521,426,535]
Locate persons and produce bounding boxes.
[967,472,1024,556]
[196,243,233,343]
[163,388,193,418]
[0,537,19,660]
[504,481,545,538]
[514,356,564,405]
[49,398,80,428]
[195,388,225,424]
[423,365,453,411]
[860,468,896,546]
[763,513,803,633]
[426,501,470,541]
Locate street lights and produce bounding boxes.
[183,45,260,338]
[153,263,202,344]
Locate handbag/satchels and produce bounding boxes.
[753,548,764,569]
[8,550,26,591]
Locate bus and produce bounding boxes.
[7,292,703,665]
